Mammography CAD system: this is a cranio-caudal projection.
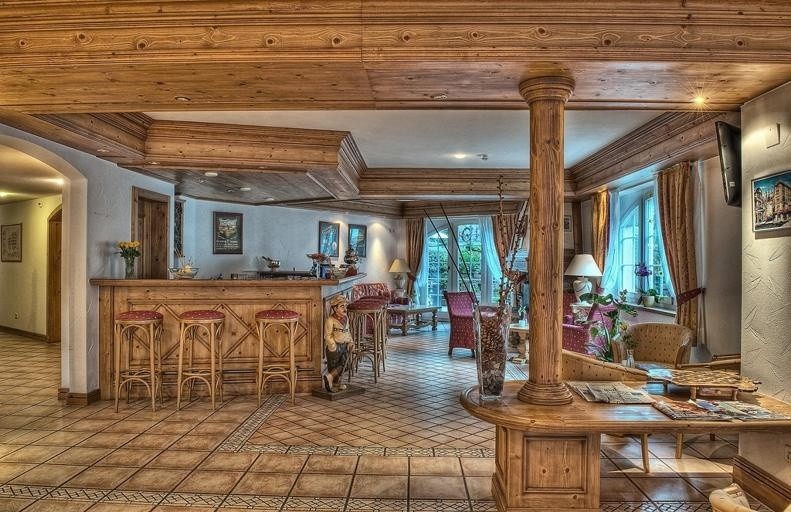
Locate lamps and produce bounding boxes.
[564,254,604,305]
[389,259,412,289]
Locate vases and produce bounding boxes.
[641,296,654,307]
[125,257,135,279]
[625,350,635,368]
[470,303,510,401]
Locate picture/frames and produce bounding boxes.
[347,224,366,257]
[0,222,23,262]
[318,221,340,257]
[564,215,572,233]
[750,169,791,232]
[212,211,243,255]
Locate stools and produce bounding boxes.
[345,295,389,383]
[177,310,225,411]
[114,311,163,413]
[254,310,299,407]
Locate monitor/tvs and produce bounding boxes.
[716,121,743,208]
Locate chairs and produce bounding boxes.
[561,349,651,474]
[611,321,692,389]
[563,293,577,325]
[675,353,741,459]
[708,483,791,512]
[441,290,500,357]
[562,301,625,359]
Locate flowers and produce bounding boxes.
[112,240,142,277]
[620,324,639,355]
[635,267,664,305]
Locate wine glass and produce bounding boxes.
[267,259,280,273]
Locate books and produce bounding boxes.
[651,398,734,421]
[707,399,791,422]
[565,379,659,405]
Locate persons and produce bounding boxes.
[321,294,353,393]
[223,223,234,238]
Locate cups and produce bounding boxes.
[518,320,524,326]
[286,276,300,280]
[325,272,330,280]
[230,273,238,280]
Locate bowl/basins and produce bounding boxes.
[333,271,346,279]
[168,267,199,280]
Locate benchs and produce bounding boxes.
[352,283,409,334]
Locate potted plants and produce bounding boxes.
[518,306,527,326]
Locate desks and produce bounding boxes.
[391,289,406,298]
[508,324,529,364]
[570,304,592,313]
